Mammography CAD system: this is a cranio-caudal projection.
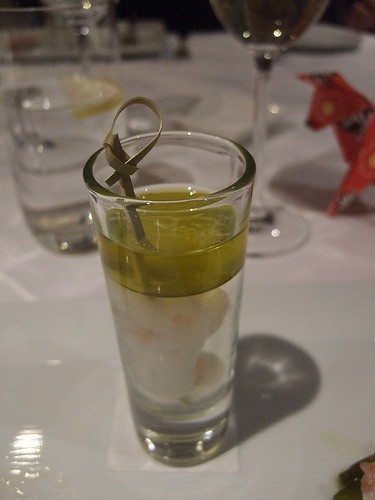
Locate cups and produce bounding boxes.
[83,131,256,467]
[0,0,125,253]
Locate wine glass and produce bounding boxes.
[208,0,328,255]
